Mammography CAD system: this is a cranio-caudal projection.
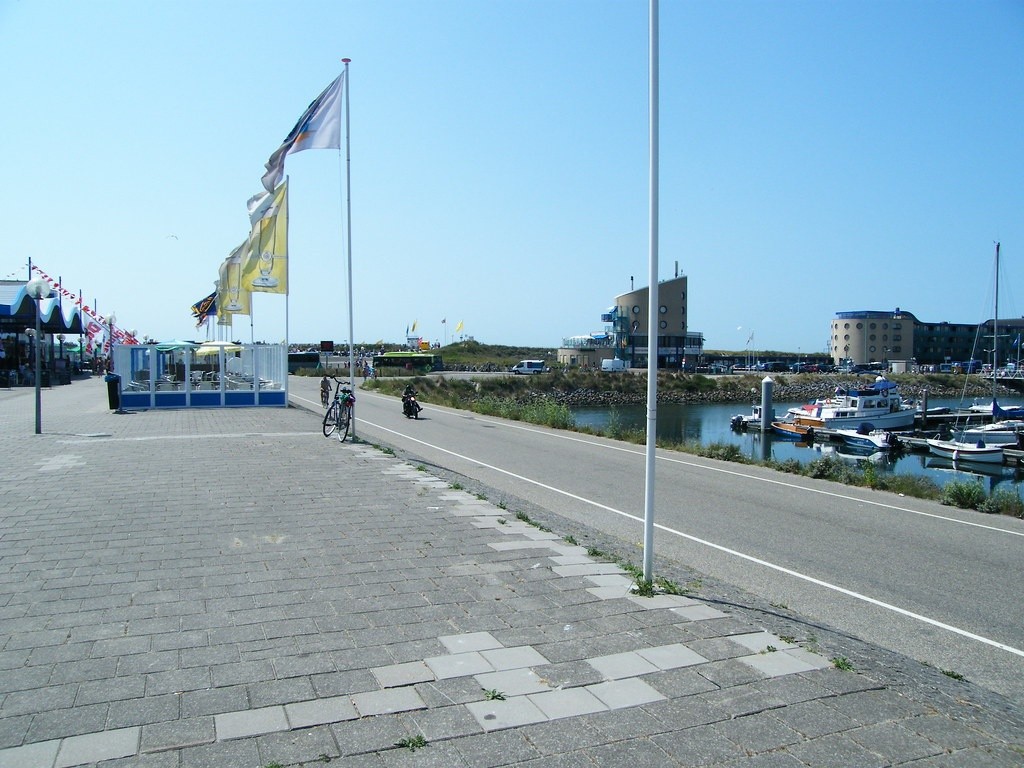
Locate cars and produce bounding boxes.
[709,361,852,373]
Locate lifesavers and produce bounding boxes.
[826,398,831,405]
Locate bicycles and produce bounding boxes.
[320,390,331,409]
[321,374,354,443]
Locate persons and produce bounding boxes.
[919,365,934,373]
[90,355,114,378]
[344,359,378,382]
[65,354,70,366]
[19,362,33,387]
[401,384,423,416]
[321,376,332,406]
[981,367,1006,377]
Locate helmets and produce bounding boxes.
[406,385,411,391]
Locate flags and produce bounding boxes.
[240,181,287,295]
[213,238,250,326]
[1012,335,1018,349]
[441,317,446,324]
[410,319,416,333]
[406,324,409,337]
[261,71,343,195]
[190,291,217,331]
[456,321,462,334]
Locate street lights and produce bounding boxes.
[57,334,65,361]
[143,334,149,345]
[28,277,52,435]
[130,329,138,344]
[104,313,116,371]
[26,328,37,377]
[78,337,85,372]
[983,349,996,372]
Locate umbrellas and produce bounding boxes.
[194,340,244,375]
[154,338,199,379]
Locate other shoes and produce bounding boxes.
[419,408,423,411]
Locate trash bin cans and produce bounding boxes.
[105,372,119,410]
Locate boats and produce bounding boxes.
[730,377,1024,467]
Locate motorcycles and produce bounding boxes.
[401,391,422,419]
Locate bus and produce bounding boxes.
[422,341,430,350]
[287,353,320,374]
[372,351,443,372]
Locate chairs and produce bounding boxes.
[128,370,282,393]
[0,366,37,386]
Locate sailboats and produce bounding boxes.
[949,240,1024,445]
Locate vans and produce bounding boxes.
[601,359,627,373]
[513,359,546,375]
